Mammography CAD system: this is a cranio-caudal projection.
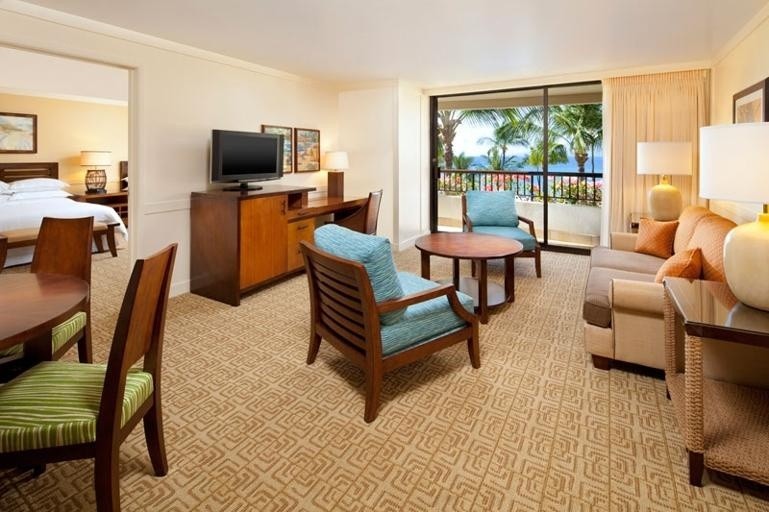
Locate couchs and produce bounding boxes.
[582,205,769,388]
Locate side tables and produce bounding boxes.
[661,275,769,486]
[628,212,648,234]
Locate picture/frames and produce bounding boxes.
[294,127,320,172]
[0,112,37,153]
[261,125,293,174]
[732,76,769,123]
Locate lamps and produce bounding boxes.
[699,122,769,313]
[79,144,112,194]
[323,149,348,198]
[636,141,692,221]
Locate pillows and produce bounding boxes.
[635,216,679,259]
[653,248,704,284]
[0,178,74,201]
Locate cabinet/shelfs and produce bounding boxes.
[190,185,369,306]
[75,191,128,227]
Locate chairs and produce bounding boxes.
[0,217,94,366]
[461,190,543,278]
[299,223,480,422]
[325,189,382,235]
[0,241,178,512]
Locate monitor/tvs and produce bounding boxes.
[210,129,285,190]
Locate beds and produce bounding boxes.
[0,161,127,268]
